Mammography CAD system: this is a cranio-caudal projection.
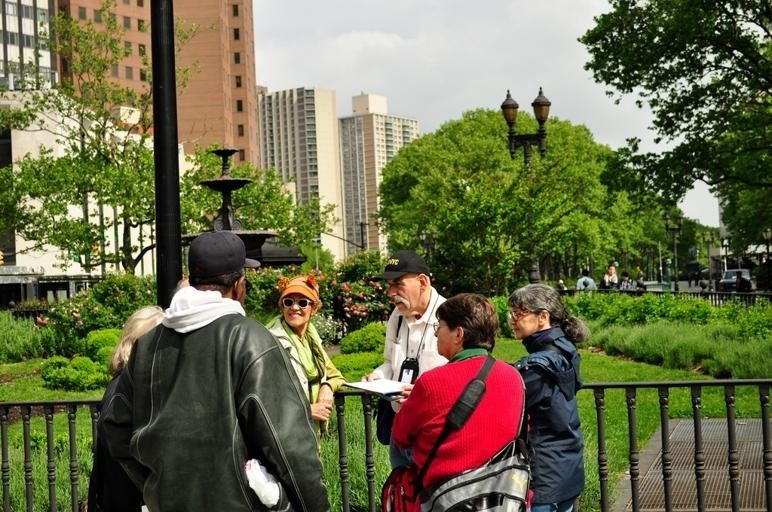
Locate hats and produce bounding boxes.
[279,276,320,316]
[370,250,430,282]
[189,230,261,277]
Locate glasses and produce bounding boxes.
[509,310,531,321]
[283,297,312,308]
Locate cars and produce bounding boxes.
[719,268,753,294]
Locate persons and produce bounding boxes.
[87,306,165,512]
[361,250,448,472]
[557,264,644,296]
[685,256,756,292]
[266,276,346,439]
[505,284,586,511]
[391,293,526,512]
[88,231,330,511]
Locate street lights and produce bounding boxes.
[661,210,685,292]
[354,219,371,250]
[502,86,553,281]
[702,230,715,291]
[720,235,731,270]
[761,227,772,290]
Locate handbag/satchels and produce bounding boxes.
[377,399,394,445]
[419,440,531,512]
[382,466,420,512]
